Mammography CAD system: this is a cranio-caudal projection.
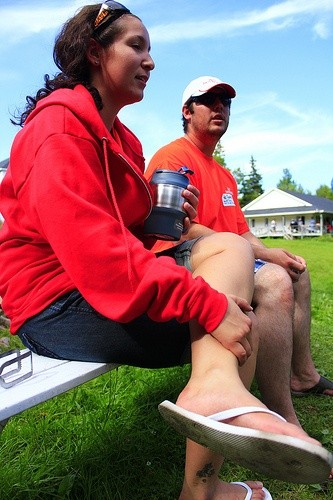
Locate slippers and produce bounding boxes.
[158,399,333,484]
[290,374,333,398]
[213,482,272,500]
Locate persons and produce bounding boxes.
[290,217,298,233]
[309,216,316,233]
[270,217,276,232]
[0,0,333,500]
[144,77,333,431]
[298,218,303,232]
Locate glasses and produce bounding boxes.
[187,92,231,106]
[93,0,130,30]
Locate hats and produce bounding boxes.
[182,76,236,106]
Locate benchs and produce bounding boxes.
[0,346,119,436]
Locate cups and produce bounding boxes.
[143,167,195,242]
